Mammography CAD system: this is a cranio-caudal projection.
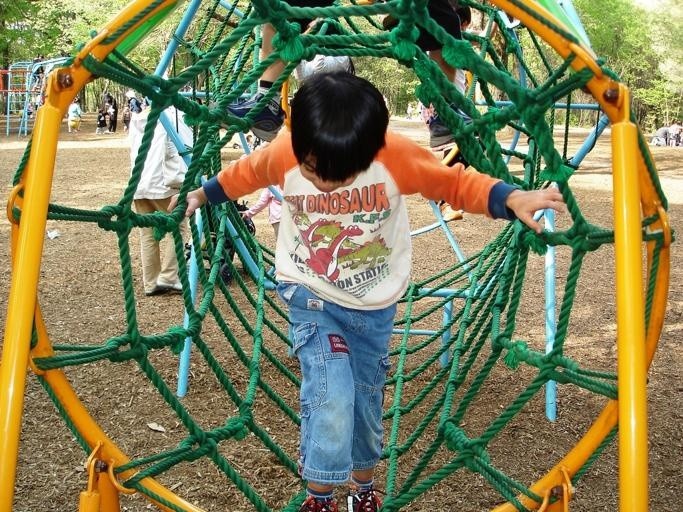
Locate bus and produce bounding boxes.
[180,92,254,149]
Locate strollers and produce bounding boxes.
[185,200,255,285]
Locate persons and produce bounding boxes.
[15,49,149,135]
[406,102,413,120]
[127,72,198,297]
[237,183,282,240]
[292,16,357,87]
[421,0,502,224]
[416,97,423,120]
[649,119,683,148]
[167,68,569,511]
[224,1,474,149]
[382,94,388,111]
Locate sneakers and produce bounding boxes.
[156,274,183,291]
[429,106,477,149]
[224,90,287,141]
[351,491,383,511]
[297,494,339,512]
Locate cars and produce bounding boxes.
[645,128,681,146]
